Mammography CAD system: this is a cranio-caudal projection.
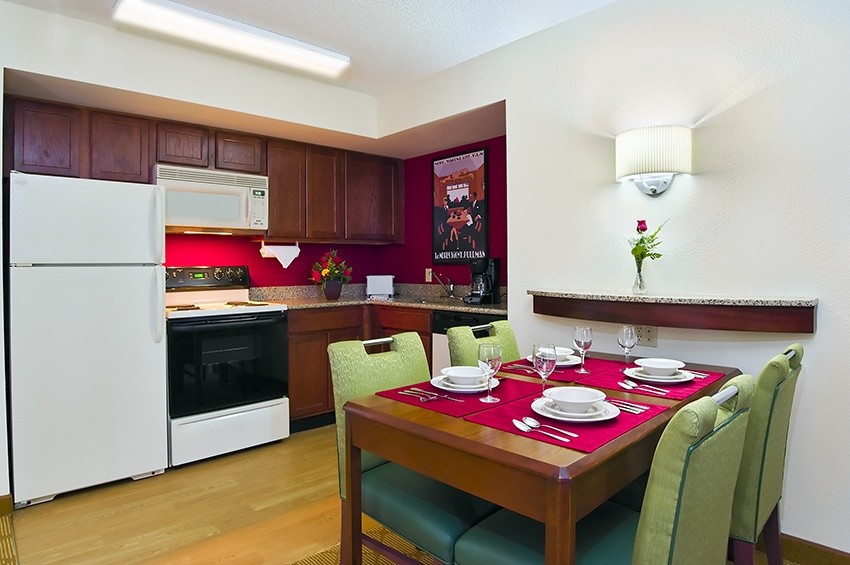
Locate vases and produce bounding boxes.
[632,272,649,296]
[323,280,343,300]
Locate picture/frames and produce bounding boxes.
[432,144,490,264]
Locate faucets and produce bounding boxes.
[428,271,456,299]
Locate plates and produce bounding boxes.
[429,374,500,394]
[622,367,695,384]
[531,397,620,421]
[526,353,583,367]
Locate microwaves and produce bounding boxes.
[151,163,269,233]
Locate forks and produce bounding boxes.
[397,389,439,402]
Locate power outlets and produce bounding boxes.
[425,268,433,283]
[634,324,658,349]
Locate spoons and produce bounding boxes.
[522,417,579,438]
[617,379,669,395]
[512,419,571,443]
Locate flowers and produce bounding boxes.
[628,217,673,271]
[306,247,352,288]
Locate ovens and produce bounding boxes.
[166,316,291,467]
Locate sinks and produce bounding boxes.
[399,298,456,305]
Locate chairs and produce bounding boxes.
[611,345,804,565]
[448,321,520,368]
[326,330,503,565]
[455,374,756,565]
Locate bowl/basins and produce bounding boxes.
[542,386,607,414]
[440,366,486,385]
[538,346,575,361]
[633,356,686,376]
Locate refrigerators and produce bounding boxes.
[6,171,170,511]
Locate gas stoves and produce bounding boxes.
[163,266,289,322]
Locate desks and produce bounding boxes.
[344,350,744,565]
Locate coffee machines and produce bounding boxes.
[463,256,502,304]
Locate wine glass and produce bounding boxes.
[616,324,638,373]
[572,325,591,375]
[532,343,557,401]
[477,341,502,403]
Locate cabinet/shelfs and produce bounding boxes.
[160,120,264,171]
[289,305,365,423]
[265,142,344,240]
[370,305,431,369]
[343,152,403,243]
[14,100,152,182]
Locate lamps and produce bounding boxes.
[112,0,352,78]
[614,126,694,196]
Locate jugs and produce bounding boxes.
[471,274,492,295]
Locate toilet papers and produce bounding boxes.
[260,245,301,269]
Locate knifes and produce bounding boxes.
[410,387,464,403]
